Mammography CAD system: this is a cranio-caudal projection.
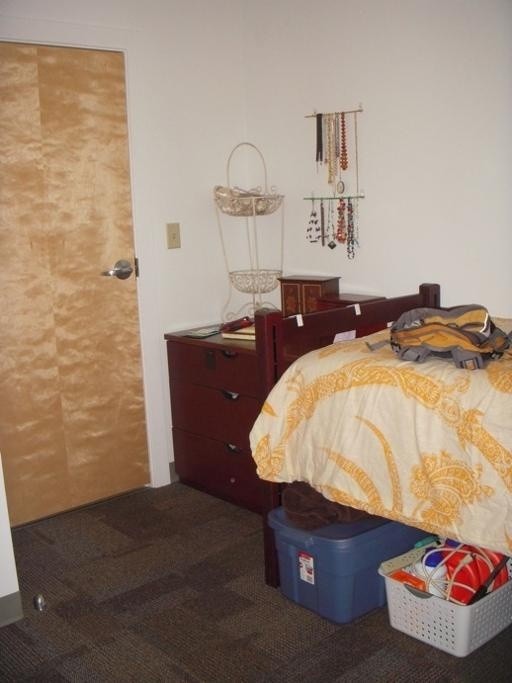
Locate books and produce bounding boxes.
[222,321,256,341]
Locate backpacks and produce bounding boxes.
[388,302,512,368]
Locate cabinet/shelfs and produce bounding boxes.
[163,315,270,516]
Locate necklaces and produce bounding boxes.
[304,109,359,261]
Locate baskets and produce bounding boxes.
[230,270,281,294]
[213,186,284,216]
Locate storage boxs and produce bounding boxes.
[265,505,433,626]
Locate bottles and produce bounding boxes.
[416,546,447,599]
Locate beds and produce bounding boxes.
[255,284,512,587]
